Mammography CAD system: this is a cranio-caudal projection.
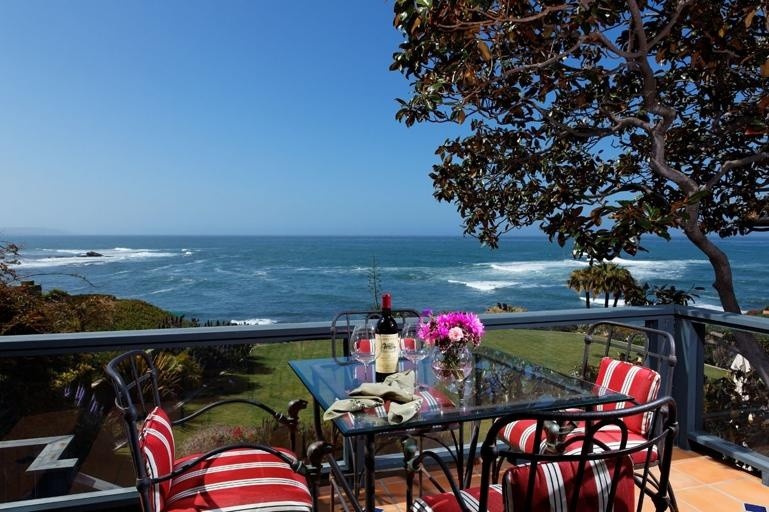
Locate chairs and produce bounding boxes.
[399,396,677,511]
[493,354,668,511]
[327,310,443,510]
[108,347,340,511]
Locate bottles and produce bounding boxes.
[375,293,401,384]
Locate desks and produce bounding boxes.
[286,345,633,512]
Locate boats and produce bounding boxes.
[86,251,102,257]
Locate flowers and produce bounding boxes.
[418,312,485,355]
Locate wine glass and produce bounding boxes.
[349,324,374,387]
[398,322,431,389]
